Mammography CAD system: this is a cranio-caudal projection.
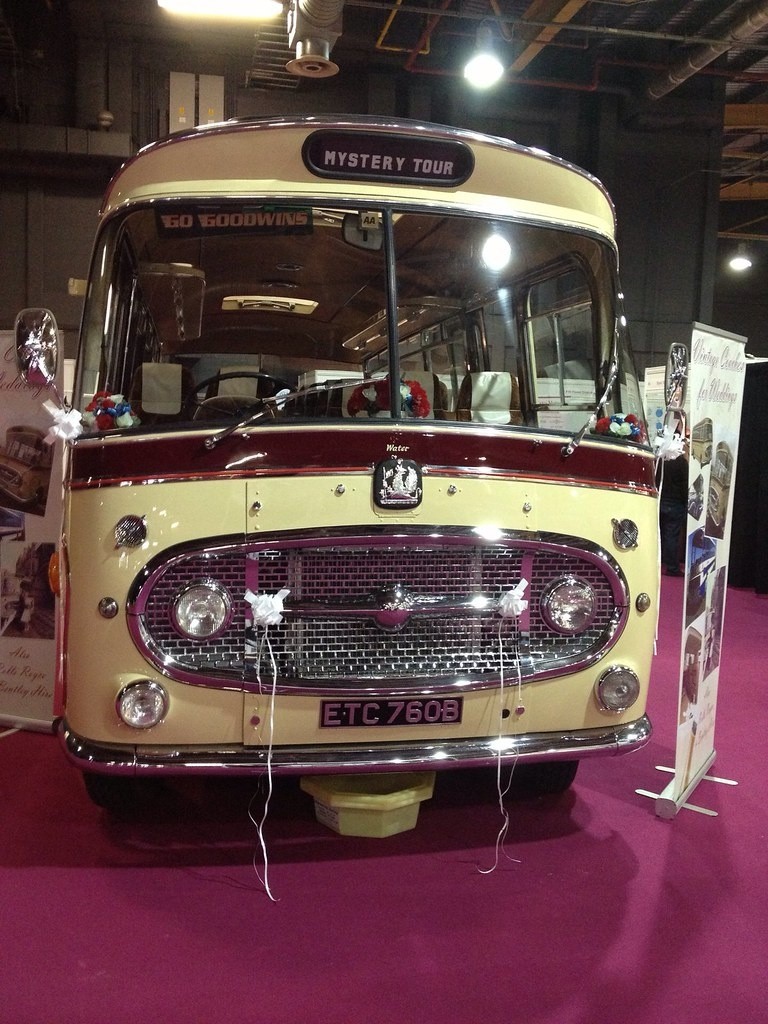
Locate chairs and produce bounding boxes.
[129,361,522,424]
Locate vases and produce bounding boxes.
[369,409,409,418]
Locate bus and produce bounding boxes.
[12,109,691,813]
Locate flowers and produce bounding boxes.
[595,412,649,442]
[344,372,431,418]
[85,390,141,428]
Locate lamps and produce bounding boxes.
[729,241,753,270]
[464,23,503,88]
